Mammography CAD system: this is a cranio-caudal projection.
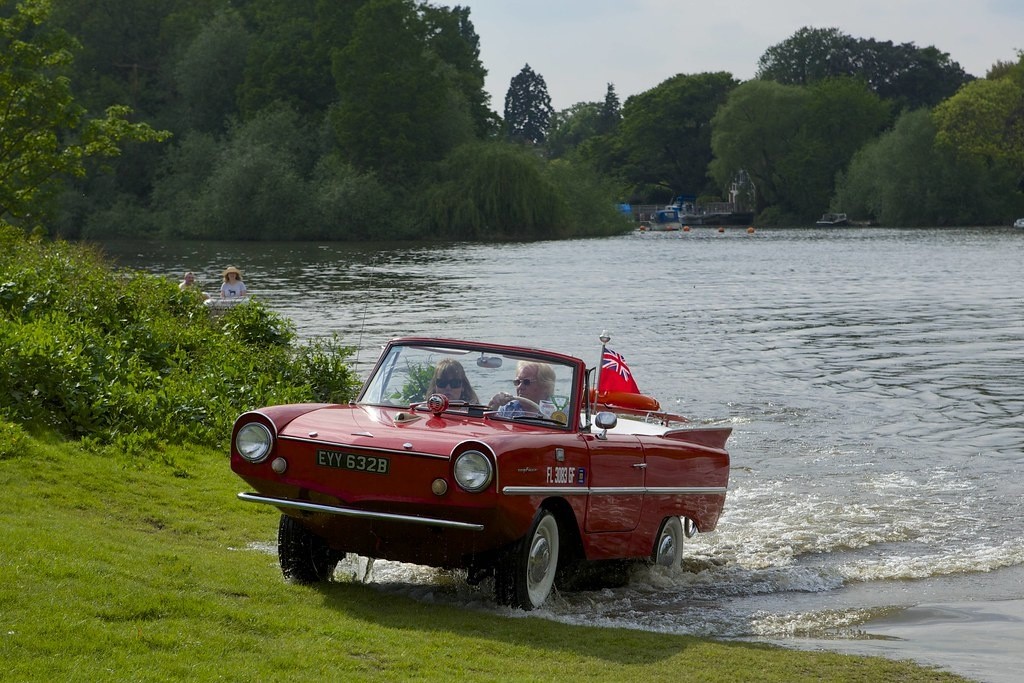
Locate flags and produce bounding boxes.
[598,348,640,394]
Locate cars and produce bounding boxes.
[230,329,733,611]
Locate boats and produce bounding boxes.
[816,213,847,228]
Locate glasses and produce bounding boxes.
[435,376,463,389]
[187,276,194,279]
[513,376,536,386]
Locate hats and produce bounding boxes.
[223,267,240,276]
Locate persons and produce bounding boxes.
[178,272,209,300]
[418,359,480,412]
[484,360,556,425]
[220,267,246,297]
